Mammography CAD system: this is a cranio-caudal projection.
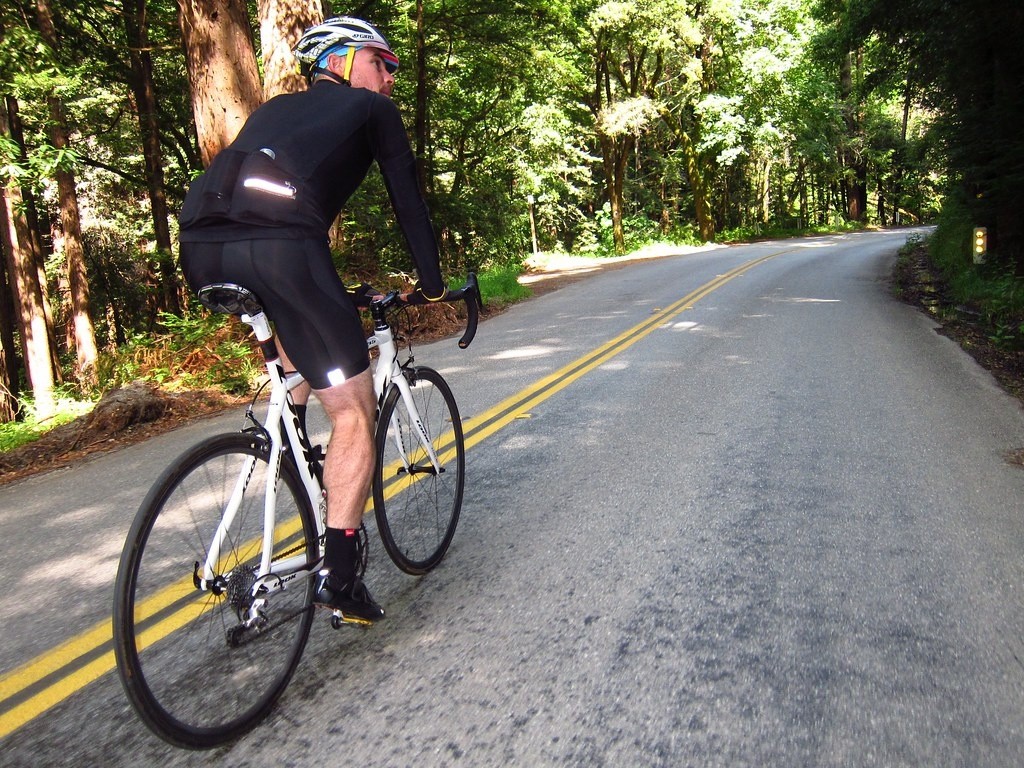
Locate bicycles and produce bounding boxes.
[111,271,491,752]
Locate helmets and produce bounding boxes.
[291,17,398,77]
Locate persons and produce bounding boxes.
[176,15,448,624]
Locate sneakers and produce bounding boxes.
[312,567,385,621]
[283,443,313,478]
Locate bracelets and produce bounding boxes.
[422,287,446,302]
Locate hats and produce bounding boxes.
[313,43,398,76]
